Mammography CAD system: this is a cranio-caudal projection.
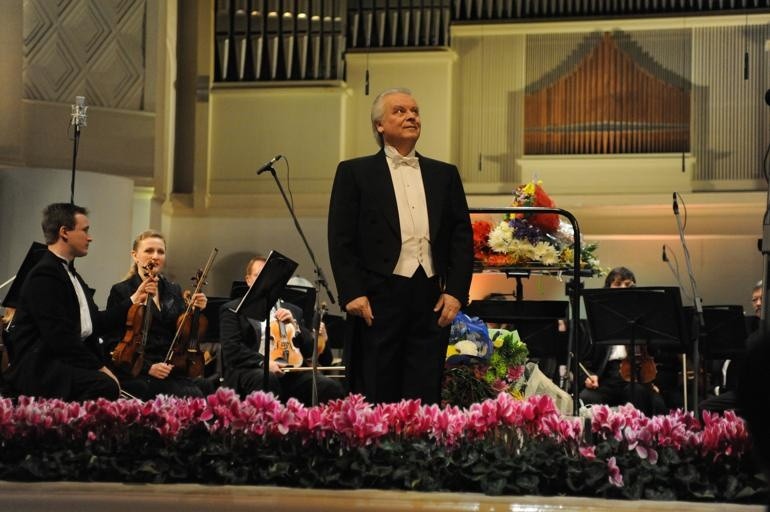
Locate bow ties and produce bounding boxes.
[391,155,419,168]
[59,256,76,277]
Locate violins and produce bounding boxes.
[269,299,303,372]
[619,344,658,384]
[317,301,327,357]
[167,268,208,381]
[112,259,156,376]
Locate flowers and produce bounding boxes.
[438,316,532,410]
[464,186,596,269]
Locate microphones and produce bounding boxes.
[255,152,283,175]
[73,94,86,126]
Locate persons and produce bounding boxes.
[12,201,118,403]
[324,87,474,409]
[580,264,669,418]
[745,279,763,337]
[104,230,209,404]
[219,255,346,408]
